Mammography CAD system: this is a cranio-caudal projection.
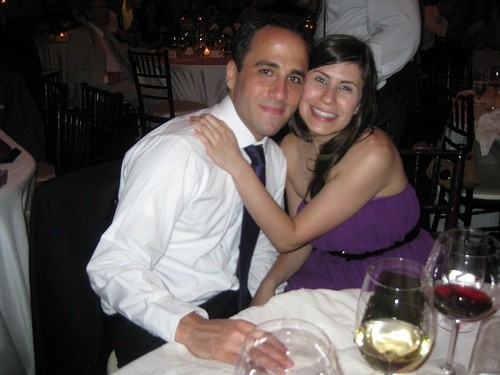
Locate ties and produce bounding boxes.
[234,146,267,313]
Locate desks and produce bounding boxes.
[111,282,500,375]
[130,47,228,111]
[0,127,37,375]
[425,88,500,234]
[33,36,69,95]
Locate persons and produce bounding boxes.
[64,0,168,115]
[135,0,173,53]
[314,0,420,169]
[446,0,500,70]
[422,0,456,51]
[105,0,141,57]
[86,19,314,375]
[189,35,435,307]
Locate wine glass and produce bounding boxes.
[484,64,500,110]
[424,230,500,375]
[166,31,242,58]
[421,232,476,333]
[472,65,490,106]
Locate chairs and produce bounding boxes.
[126,47,209,139]
[426,85,500,237]
[399,143,469,255]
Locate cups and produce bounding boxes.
[352,257,436,375]
[232,317,341,375]
[466,315,500,374]
